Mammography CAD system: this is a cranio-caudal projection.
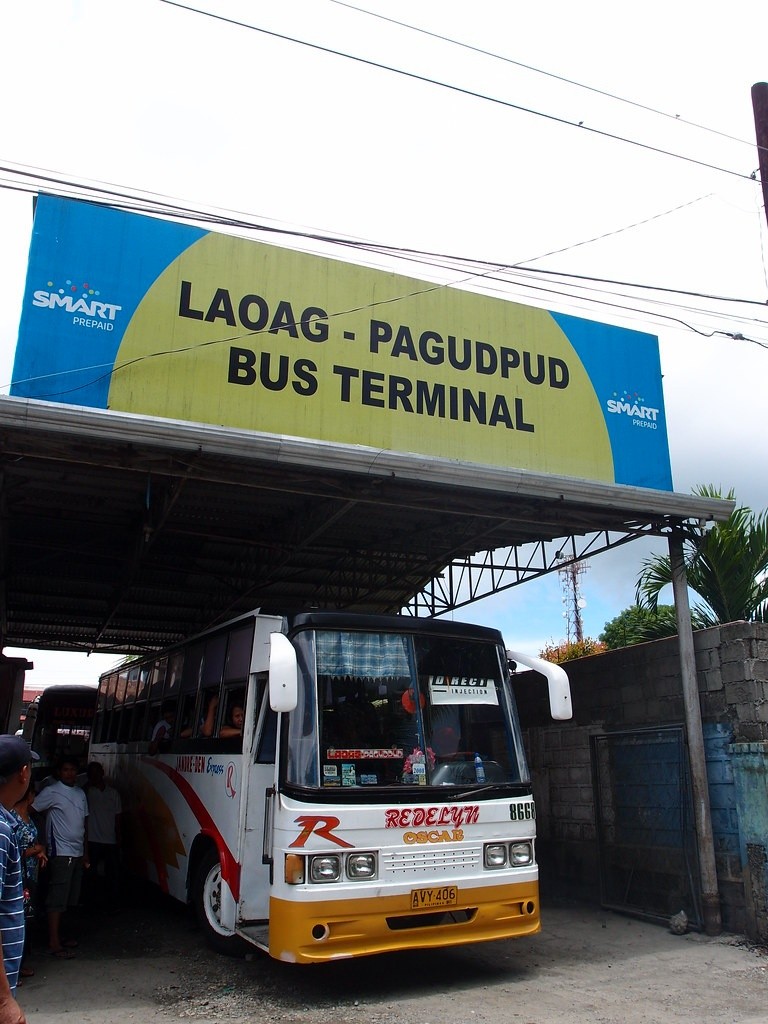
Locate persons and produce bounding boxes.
[433,727,459,765]
[0,733,41,1024]
[151,694,245,742]
[16,755,122,986]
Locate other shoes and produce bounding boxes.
[20,967,34,977]
[61,939,78,946]
[46,948,75,958]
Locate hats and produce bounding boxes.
[0,734,40,775]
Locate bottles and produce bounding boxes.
[473,753,487,784]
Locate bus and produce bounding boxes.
[29,685,99,783]
[85,604,572,966]
